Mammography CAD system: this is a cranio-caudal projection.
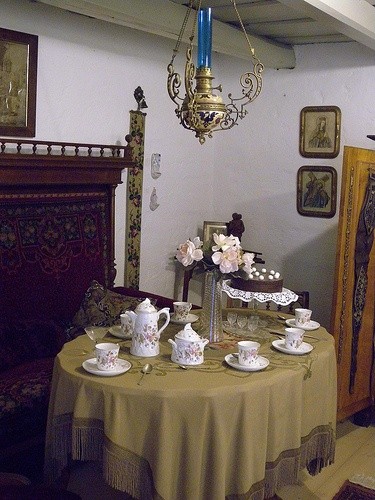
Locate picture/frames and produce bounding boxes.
[300,105,341,159]
[0,27,38,138]
[297,166,338,219]
[203,220,228,243]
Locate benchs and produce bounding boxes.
[0,135,201,459]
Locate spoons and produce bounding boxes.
[137,363,152,385]
[278,336,309,343]
[277,314,288,320]
[178,364,211,371]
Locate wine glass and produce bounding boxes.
[226,310,259,335]
[84,326,109,357]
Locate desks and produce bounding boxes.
[44,309,338,500]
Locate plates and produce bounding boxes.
[108,325,134,339]
[285,318,321,330]
[163,313,199,324]
[271,339,314,354]
[224,353,270,371]
[82,357,131,376]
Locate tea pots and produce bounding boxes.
[168,323,209,365]
[124,298,170,358]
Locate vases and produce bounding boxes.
[199,272,224,343]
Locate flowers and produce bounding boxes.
[174,233,256,284]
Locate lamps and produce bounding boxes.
[167,0,265,146]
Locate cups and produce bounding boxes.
[285,328,305,350]
[295,308,312,327]
[172,301,192,320]
[237,340,260,366]
[94,343,120,371]
[120,313,135,335]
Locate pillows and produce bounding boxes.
[59,278,157,350]
